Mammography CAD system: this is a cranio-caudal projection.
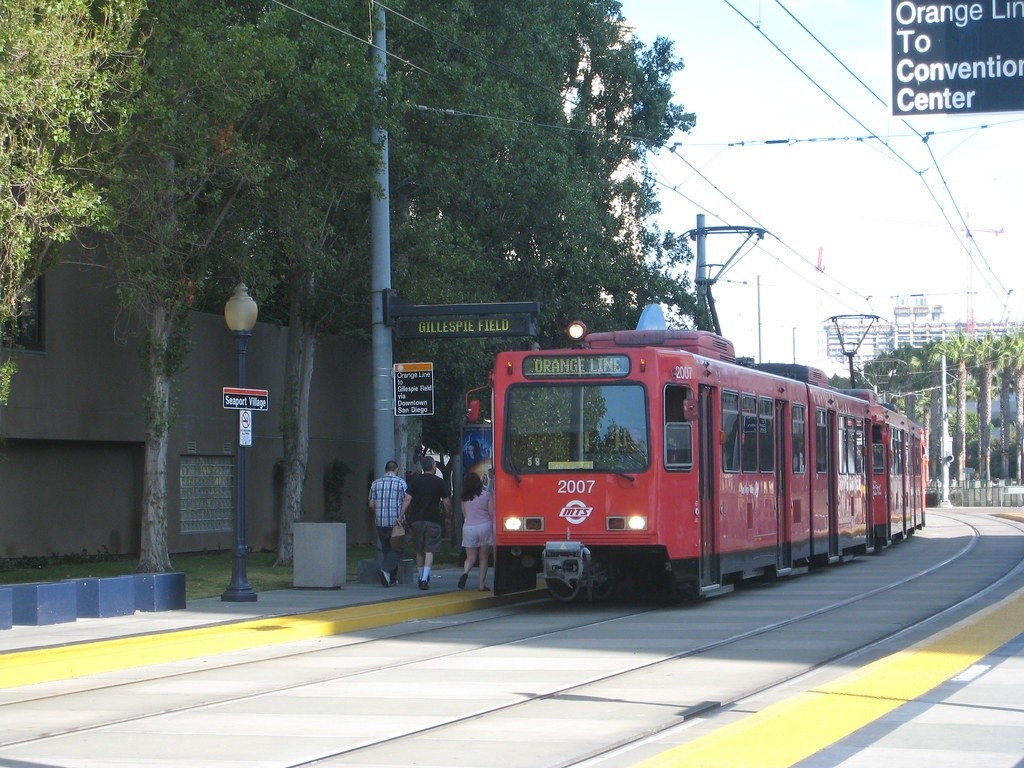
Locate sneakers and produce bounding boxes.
[377,569,399,588]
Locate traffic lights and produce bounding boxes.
[945,454,955,463]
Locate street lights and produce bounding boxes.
[221,283,258,601]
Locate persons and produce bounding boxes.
[464,439,495,502]
[368,461,408,587]
[396,457,452,590]
[457,471,495,592]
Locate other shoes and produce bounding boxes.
[417,580,428,589]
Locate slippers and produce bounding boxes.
[458,573,468,589]
[480,588,490,591]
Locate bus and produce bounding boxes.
[465,304,926,606]
